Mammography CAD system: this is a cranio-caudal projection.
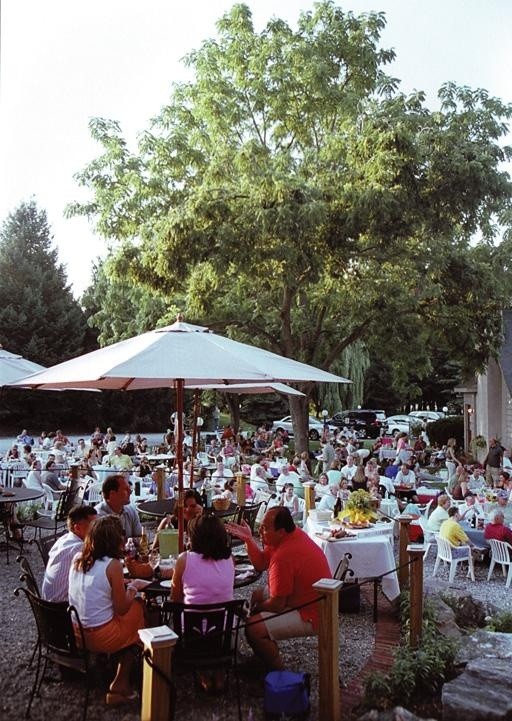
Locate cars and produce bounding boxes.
[387,415,425,437]
[273,415,337,442]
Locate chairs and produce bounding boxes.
[15,587,121,721]
[14,556,60,698]
[161,600,249,720]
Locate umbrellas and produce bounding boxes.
[171,381,308,489]
[5,311,353,555]
[0,344,102,390]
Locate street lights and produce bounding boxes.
[196,417,204,451]
[442,407,448,415]
[322,410,328,423]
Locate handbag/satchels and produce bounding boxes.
[262,670,312,721]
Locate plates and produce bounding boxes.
[234,564,255,571]
[159,579,172,588]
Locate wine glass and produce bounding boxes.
[183,532,190,550]
[168,553,178,568]
[148,552,161,582]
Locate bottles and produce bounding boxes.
[125,538,135,563]
[139,525,148,563]
[199,489,206,511]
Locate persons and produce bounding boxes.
[40,503,97,602]
[224,505,332,699]
[168,512,239,698]
[1,412,511,560]
[67,515,153,705]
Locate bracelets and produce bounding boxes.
[126,584,140,593]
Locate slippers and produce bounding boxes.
[105,690,140,706]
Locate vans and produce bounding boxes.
[325,410,388,438]
[408,411,444,422]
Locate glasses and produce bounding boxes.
[121,529,126,536]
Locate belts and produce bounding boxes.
[73,617,114,632]
